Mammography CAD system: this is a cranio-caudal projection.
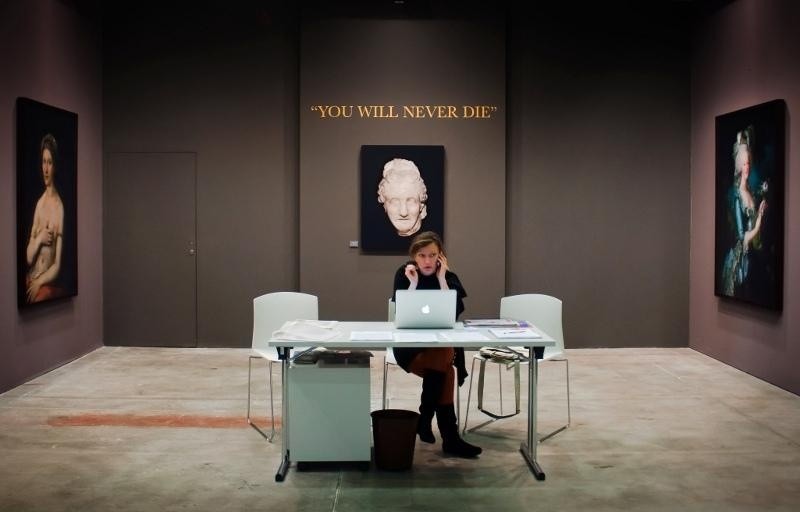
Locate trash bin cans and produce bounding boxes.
[370,409,420,473]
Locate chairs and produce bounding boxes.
[378,293,460,439]
[247,291,320,443]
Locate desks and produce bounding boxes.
[267,320,556,481]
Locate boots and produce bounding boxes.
[434,402,483,459]
[415,366,449,445]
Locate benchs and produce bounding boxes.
[463,294,571,443]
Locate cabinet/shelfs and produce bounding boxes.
[288,349,372,471]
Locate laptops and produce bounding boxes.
[396,289,457,329]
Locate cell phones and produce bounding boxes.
[436,256,447,267]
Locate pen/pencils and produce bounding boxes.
[505,331,526,334]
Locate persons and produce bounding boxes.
[719,131,768,297]
[377,157,428,237]
[391,232,483,459]
[26,134,65,303]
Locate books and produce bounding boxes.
[463,319,528,328]
[488,329,542,339]
